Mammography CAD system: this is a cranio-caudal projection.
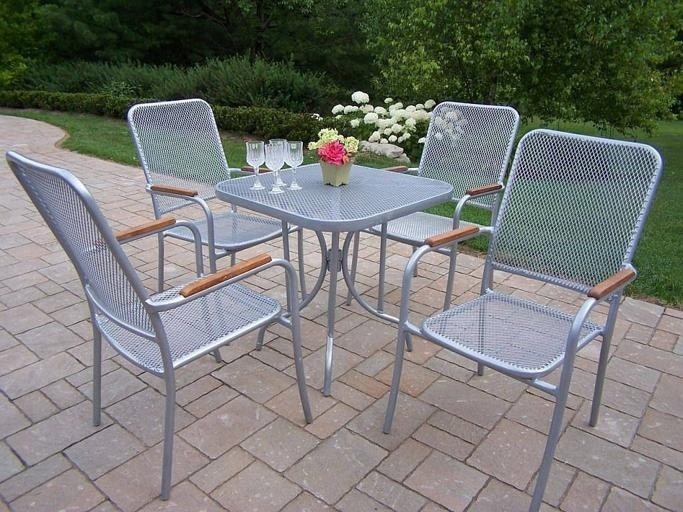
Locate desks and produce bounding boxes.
[214,162,454,398]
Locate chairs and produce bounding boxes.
[381,126,663,511]
[127,96,305,348]
[346,101,519,341]
[6,150,317,502]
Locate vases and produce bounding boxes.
[319,159,353,186]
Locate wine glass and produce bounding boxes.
[245,137,305,196]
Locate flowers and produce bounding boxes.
[311,96,470,152]
[307,128,359,168]
